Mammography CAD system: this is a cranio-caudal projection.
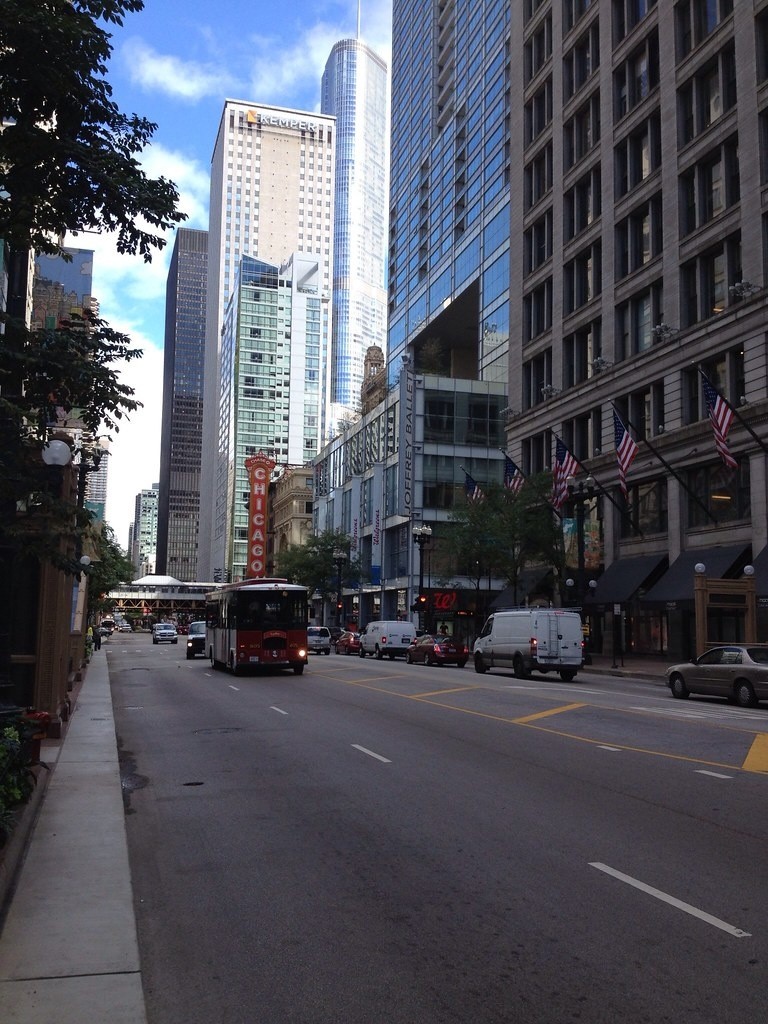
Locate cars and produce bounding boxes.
[97,618,133,638]
[663,644,768,708]
[404,633,470,669]
[329,627,362,655]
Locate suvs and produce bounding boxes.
[186,621,206,659]
[307,626,332,655]
[152,623,178,644]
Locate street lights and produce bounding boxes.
[565,471,598,666]
[412,524,433,635]
[333,551,347,626]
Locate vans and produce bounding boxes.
[472,606,585,682]
[358,620,417,660]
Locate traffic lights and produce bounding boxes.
[414,594,426,611]
[336,601,343,615]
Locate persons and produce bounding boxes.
[440,622,449,637]
[86,624,101,651]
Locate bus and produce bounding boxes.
[203,577,317,676]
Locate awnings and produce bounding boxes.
[488,543,768,610]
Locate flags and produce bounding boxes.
[613,403,639,503]
[551,430,580,511]
[464,472,488,519]
[700,370,739,468]
[502,453,528,508]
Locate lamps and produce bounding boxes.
[695,563,706,573]
[744,565,754,575]
[729,279,751,296]
[500,407,512,416]
[652,322,669,337]
[591,357,604,367]
[81,556,90,565]
[540,384,553,394]
